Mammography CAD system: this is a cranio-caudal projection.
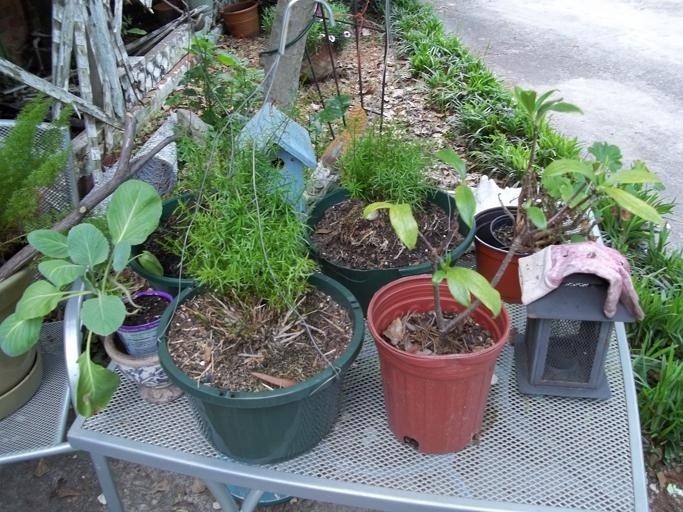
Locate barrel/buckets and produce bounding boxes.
[156,270,366,467]
[301,183,476,320]
[125,192,240,295]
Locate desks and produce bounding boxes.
[66,246,649,512]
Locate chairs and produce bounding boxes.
[0,119,124,512]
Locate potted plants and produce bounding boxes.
[219,2,262,39]
[363,81,660,455]
[129,30,259,289]
[472,198,566,305]
[150,34,370,464]
[2,177,177,389]
[297,112,479,307]
